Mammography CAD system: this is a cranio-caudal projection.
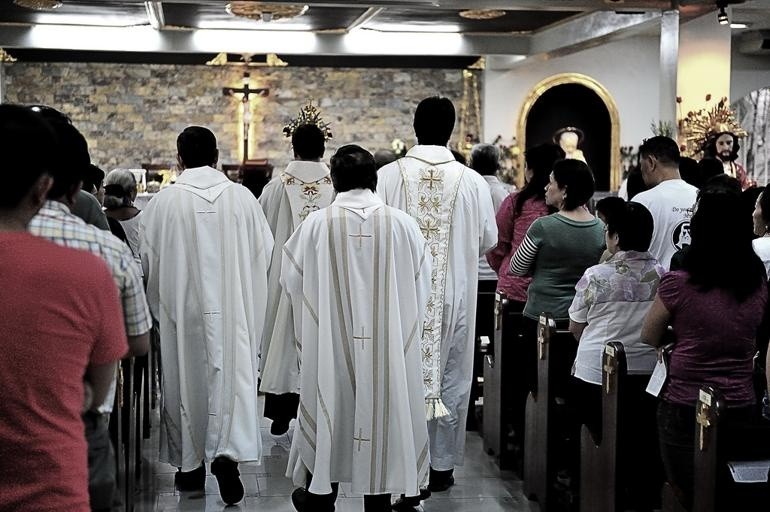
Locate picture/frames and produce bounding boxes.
[129,168,147,192]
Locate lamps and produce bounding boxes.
[718,5,729,25]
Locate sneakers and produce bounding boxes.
[269,418,291,437]
[429,476,454,491]
[174,469,204,491]
[211,456,245,504]
[291,487,333,512]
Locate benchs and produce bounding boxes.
[107,344,157,511]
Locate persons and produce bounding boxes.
[375,95,499,500]
[2,102,151,511]
[137,126,275,505]
[455,127,769,510]
[278,144,434,512]
[374,149,397,170]
[257,124,336,435]
